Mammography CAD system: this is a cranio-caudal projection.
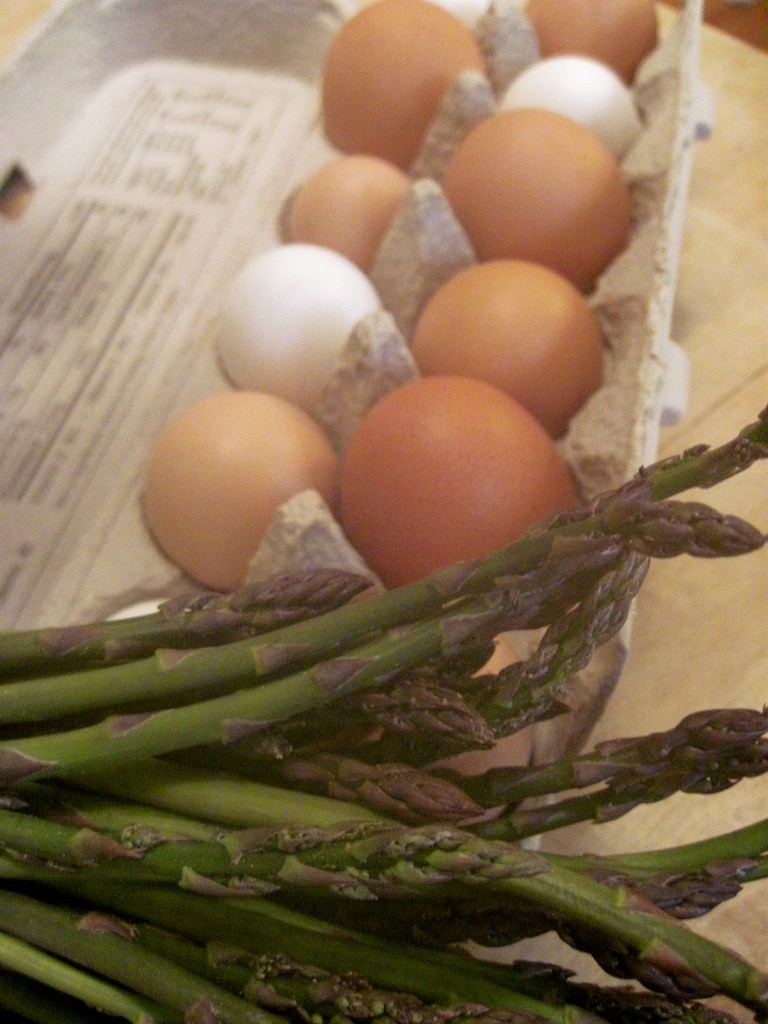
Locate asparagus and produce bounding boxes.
[0,406,768,1024]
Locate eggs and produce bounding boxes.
[138,0,657,598]
